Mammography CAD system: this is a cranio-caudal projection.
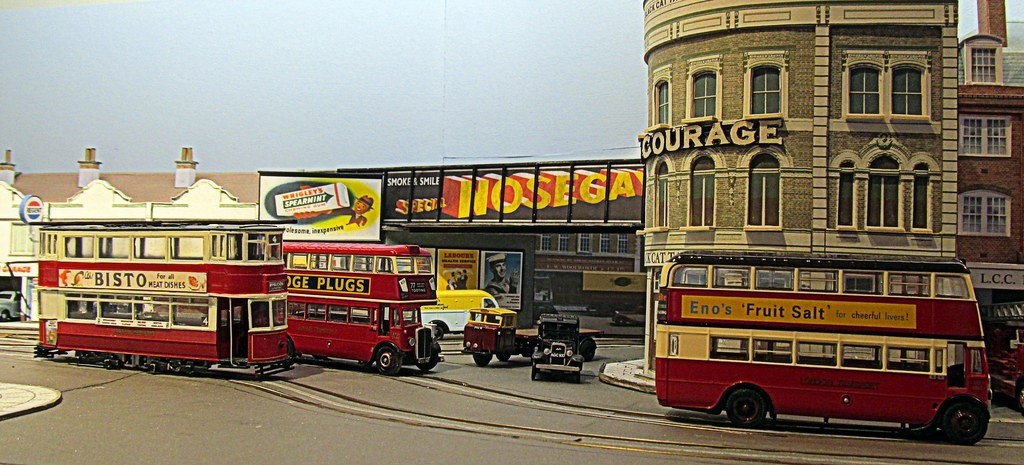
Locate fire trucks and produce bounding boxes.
[981,301,1024,413]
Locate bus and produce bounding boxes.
[283,241,445,375]
[34,219,295,380]
[654,249,992,446]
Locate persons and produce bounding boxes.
[485,254,519,295]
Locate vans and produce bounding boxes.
[0,291,22,322]
[420,289,498,341]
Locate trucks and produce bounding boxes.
[462,309,603,368]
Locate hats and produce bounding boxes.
[486,253,507,265]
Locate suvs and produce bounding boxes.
[532,313,583,383]
